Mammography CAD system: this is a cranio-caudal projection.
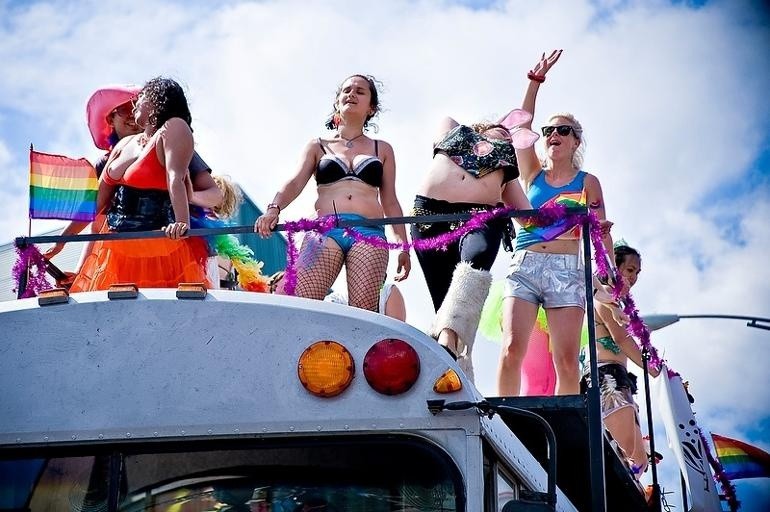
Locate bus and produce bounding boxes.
[0,202,731,512]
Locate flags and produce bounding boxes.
[28,150,98,221]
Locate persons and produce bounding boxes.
[379,273,406,322]
[211,175,240,220]
[581,241,659,482]
[254,74,411,311]
[38,72,212,292]
[180,485,345,512]
[499,47,629,398]
[479,272,630,397]
[409,106,613,387]
[55,85,222,287]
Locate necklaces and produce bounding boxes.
[137,134,151,148]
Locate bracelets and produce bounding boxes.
[401,251,410,256]
[527,71,546,84]
[593,288,597,295]
[266,203,280,214]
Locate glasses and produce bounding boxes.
[110,103,133,118]
[541,125,576,137]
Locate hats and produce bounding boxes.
[86,84,141,150]
